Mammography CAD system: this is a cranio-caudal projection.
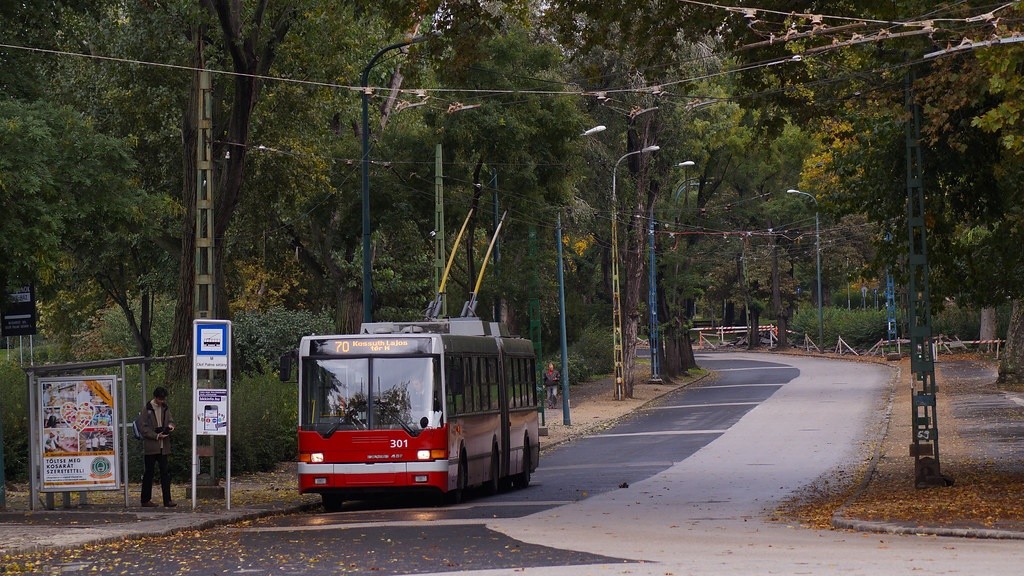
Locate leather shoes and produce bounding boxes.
[142,500,159,507]
[164,500,177,506]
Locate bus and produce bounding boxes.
[277,207,540,495]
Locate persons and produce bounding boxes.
[134,386,175,507]
[542,361,560,411]
[405,375,438,417]
[42,384,109,452]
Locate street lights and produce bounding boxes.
[647,160,701,386]
[611,143,663,406]
[786,188,828,354]
[363,32,444,327]
[482,124,607,347]
